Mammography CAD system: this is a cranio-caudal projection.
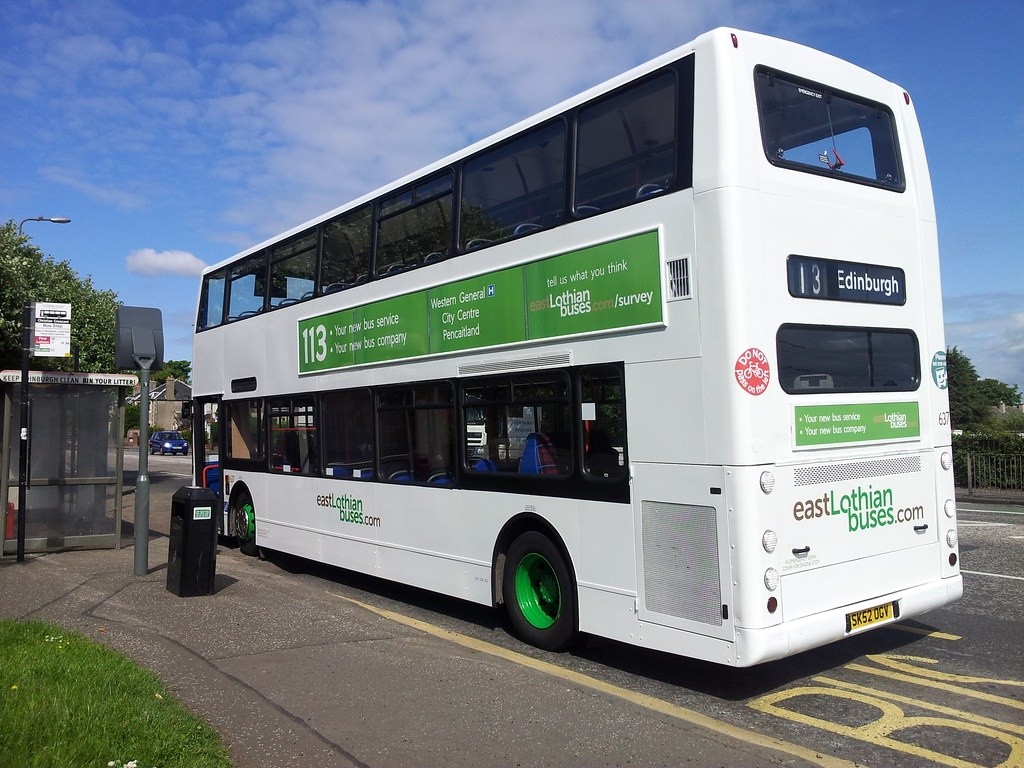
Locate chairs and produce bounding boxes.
[221,183,666,321]
[201,430,560,498]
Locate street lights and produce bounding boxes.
[18,215,74,241]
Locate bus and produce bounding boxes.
[188,30,965,672]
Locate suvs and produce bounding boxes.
[149,430,190,457]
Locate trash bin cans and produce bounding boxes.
[166,486,217,598]
